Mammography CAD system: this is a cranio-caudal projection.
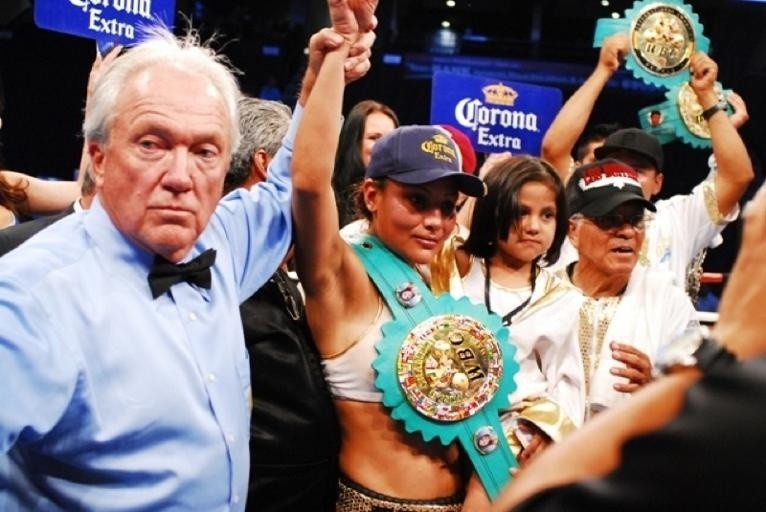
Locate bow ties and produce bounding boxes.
[147,248,216,299]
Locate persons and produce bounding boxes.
[0,0,766,512]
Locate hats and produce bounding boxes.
[594,128,665,172]
[366,124,485,198]
[565,157,657,218]
